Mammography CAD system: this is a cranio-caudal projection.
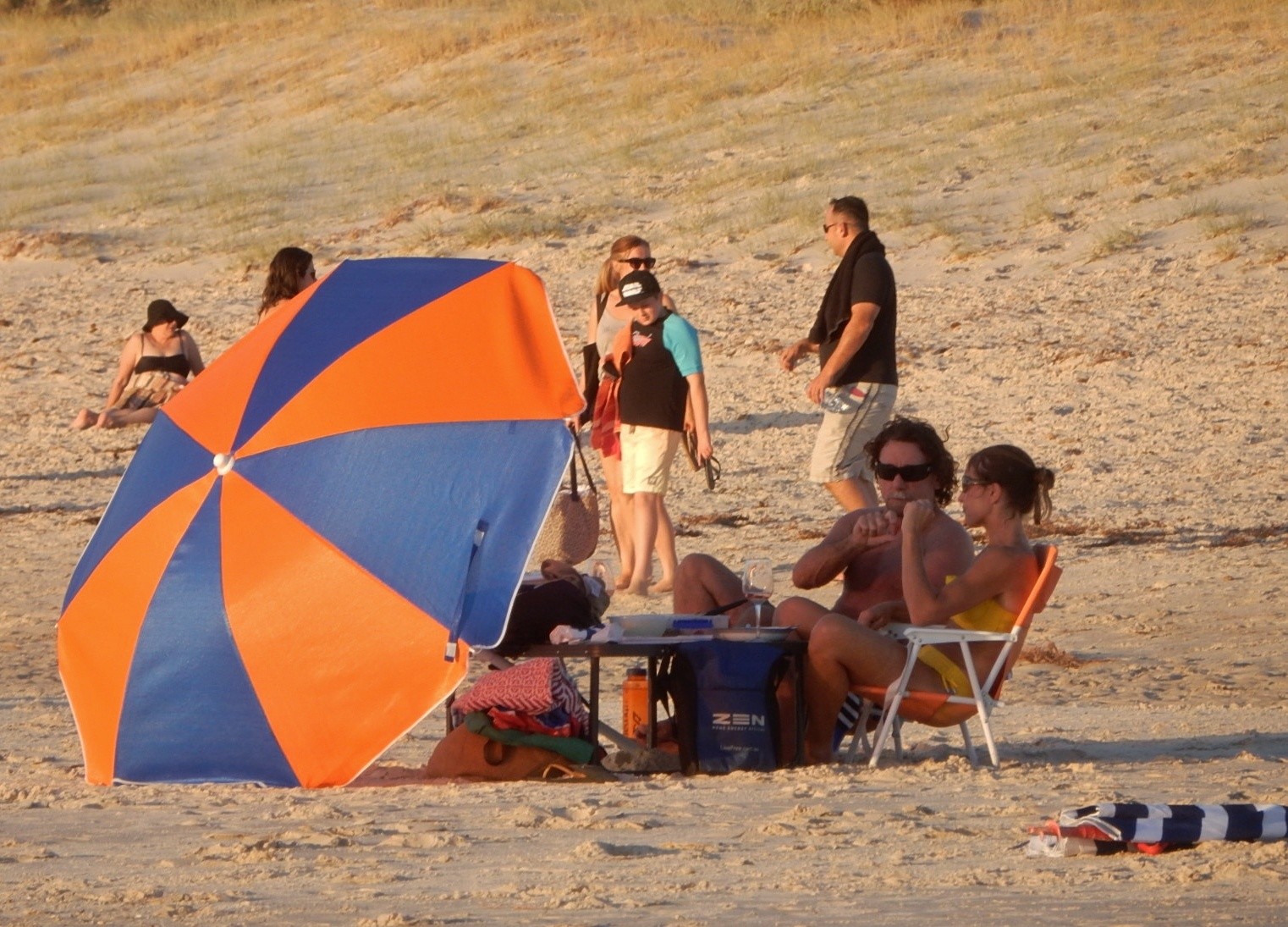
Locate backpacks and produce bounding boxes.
[491,575,610,657]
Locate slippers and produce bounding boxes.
[681,428,706,472]
[704,455,721,490]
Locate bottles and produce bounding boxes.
[804,382,844,414]
[622,666,648,745]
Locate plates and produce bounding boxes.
[709,626,799,642]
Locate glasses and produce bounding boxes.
[961,475,992,492]
[823,223,846,233]
[619,257,656,269]
[305,270,316,279]
[876,462,936,481]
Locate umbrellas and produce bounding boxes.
[57,257,588,792]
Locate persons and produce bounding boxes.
[568,235,677,588]
[257,246,317,325]
[773,445,1056,768]
[780,196,898,514]
[70,298,204,435]
[673,415,975,716]
[606,269,715,598]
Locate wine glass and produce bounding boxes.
[742,558,772,642]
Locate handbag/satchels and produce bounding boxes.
[535,483,599,569]
[424,655,604,782]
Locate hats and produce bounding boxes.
[143,300,189,332]
[616,270,661,307]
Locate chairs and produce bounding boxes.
[840,543,1065,768]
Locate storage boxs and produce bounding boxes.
[605,614,732,636]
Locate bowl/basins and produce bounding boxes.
[607,614,670,637]
[669,614,730,636]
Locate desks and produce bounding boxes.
[504,628,809,756]
